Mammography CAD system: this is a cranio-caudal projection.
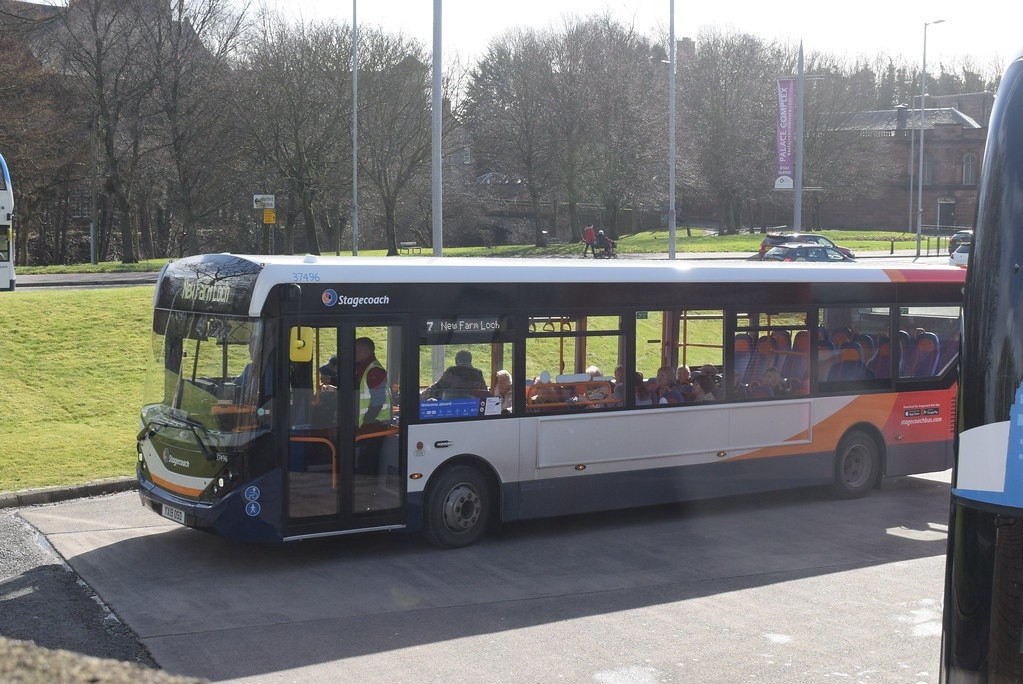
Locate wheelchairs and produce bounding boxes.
[592,238,618,259]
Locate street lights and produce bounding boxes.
[916,19,947,256]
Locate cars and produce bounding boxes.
[760,241,857,263]
[949,241,971,265]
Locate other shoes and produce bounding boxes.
[594,254,596,257]
[584,255,587,257]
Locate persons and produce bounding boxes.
[422,350,489,404]
[232,338,258,388]
[494,371,514,410]
[319,336,392,519]
[585,223,597,258]
[597,229,614,254]
[532,363,805,403]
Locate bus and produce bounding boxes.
[0,155,19,291]
[940,53,1023,684]
[137,247,969,550]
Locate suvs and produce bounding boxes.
[759,231,859,260]
[948,230,974,256]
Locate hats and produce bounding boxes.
[539,370,551,385]
[598,229,603,233]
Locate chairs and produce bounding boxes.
[724,322,941,396]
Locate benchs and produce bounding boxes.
[400,241,421,254]
